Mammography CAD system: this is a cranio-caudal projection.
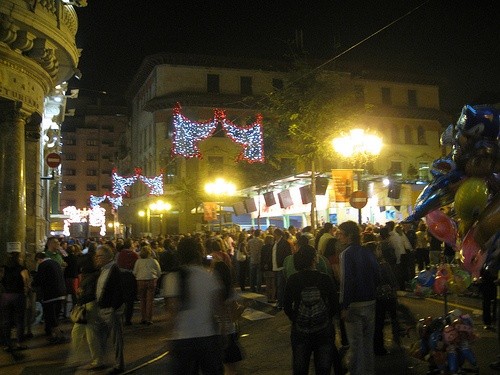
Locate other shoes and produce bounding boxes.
[267,297,284,309]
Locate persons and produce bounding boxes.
[160,226,250,375]
[284,246,338,375]
[30,234,162,375]
[336,221,376,375]
[239,220,455,318]
[0,252,34,359]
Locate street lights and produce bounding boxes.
[150,200,172,235]
[332,127,382,226]
[205,178,237,232]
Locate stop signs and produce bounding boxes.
[349,190,369,209]
[47,152,61,168]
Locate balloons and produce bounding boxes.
[403,103,500,270]
[416,309,480,372]
[410,263,474,296]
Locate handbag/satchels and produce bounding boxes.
[237,246,247,262]
[70,301,88,324]
